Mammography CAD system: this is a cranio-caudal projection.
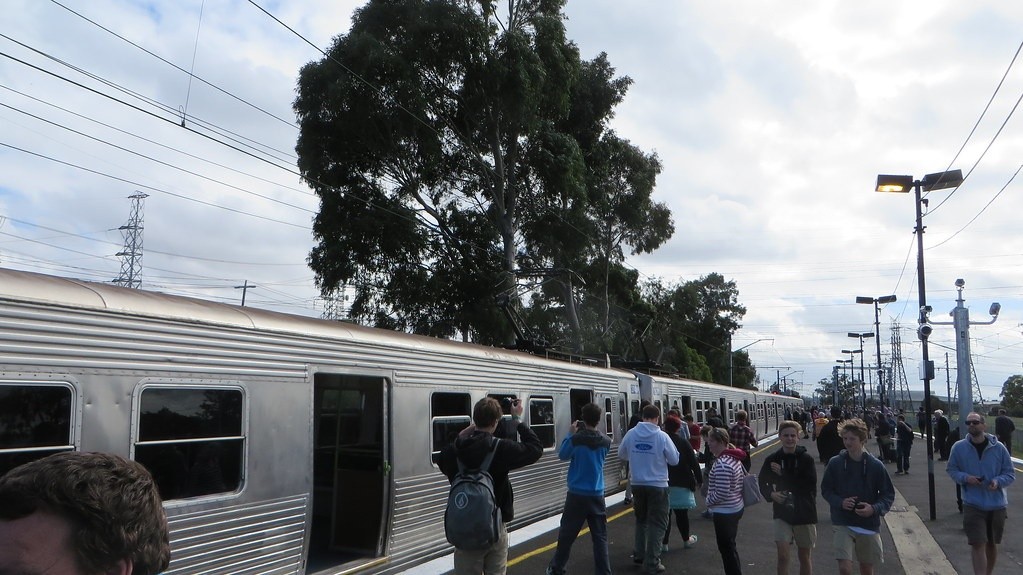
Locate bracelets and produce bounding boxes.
[513,416,521,420]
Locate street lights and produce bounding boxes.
[842,349,863,395]
[848,332,875,411]
[836,359,852,388]
[856,295,897,412]
[876,169,964,520]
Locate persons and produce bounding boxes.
[624,400,651,505]
[729,410,758,473]
[946,412,1016,575]
[817,407,845,467]
[996,410,1015,455]
[890,416,913,475]
[821,420,895,575]
[786,404,906,464]
[931,410,959,461]
[757,420,817,575]
[437,397,544,575]
[916,406,926,438]
[694,407,745,575]
[618,405,680,575]
[0,452,170,575]
[662,406,701,551]
[547,403,611,575]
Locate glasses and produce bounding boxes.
[965,420,983,425]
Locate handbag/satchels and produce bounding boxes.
[740,462,762,507]
[880,434,893,446]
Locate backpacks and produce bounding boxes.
[444,436,503,551]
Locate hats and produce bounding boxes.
[665,416,682,433]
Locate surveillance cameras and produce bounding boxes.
[921,305,932,312]
[990,303,1001,314]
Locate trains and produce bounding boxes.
[0,266,806,575]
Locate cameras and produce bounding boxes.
[577,421,585,427]
[781,490,796,511]
[853,499,865,509]
[502,396,517,406]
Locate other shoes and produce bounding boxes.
[895,470,903,474]
[546,567,553,575]
[803,435,809,439]
[649,564,665,575]
[662,543,669,552]
[623,497,633,506]
[702,509,713,518]
[683,535,697,548]
[905,469,909,474]
[633,554,644,567]
